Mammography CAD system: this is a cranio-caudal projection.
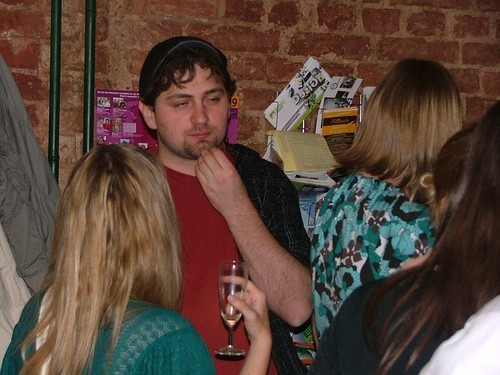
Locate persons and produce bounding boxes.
[308,58,463,345]
[376,102,500,375]
[312,123,473,375]
[1,146,272,375]
[138,35,314,375]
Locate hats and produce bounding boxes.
[138,36,227,100]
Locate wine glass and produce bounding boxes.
[215,261,249,356]
